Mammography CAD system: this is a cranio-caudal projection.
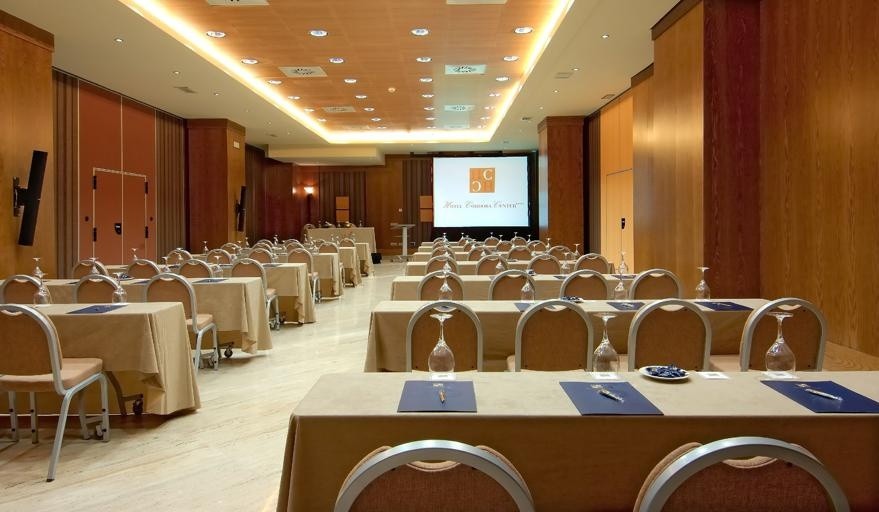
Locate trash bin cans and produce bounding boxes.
[372,253,381,264]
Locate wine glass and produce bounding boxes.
[767,309,796,377]
[590,308,620,378]
[520,267,535,302]
[28,217,365,311]
[429,311,456,381]
[611,270,629,302]
[429,230,797,273]
[436,271,452,300]
[693,266,712,301]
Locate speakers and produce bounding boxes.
[18,150,48,247]
[239,185,248,232]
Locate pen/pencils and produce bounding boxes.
[600,390,624,403]
[804,388,838,399]
[439,390,445,402]
